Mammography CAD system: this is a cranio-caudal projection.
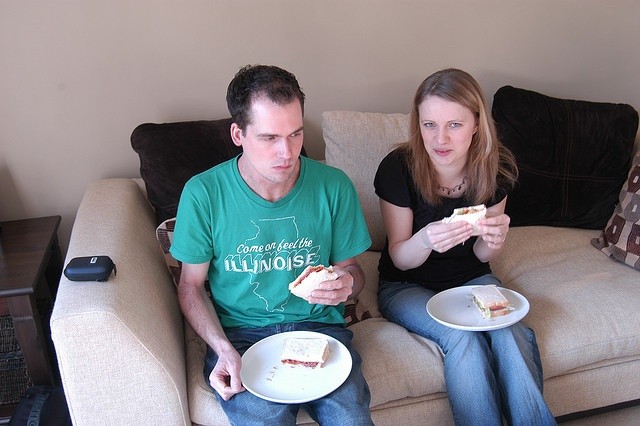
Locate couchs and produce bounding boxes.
[50,157,639,425]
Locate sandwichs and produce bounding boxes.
[288,264,341,306]
[442,204,487,236]
[280,338,330,370]
[471,287,510,320]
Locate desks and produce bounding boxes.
[0,213,65,426]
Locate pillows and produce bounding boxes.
[129,118,307,224]
[591,145,639,270]
[491,83,640,230]
[153,217,212,298]
[321,110,412,251]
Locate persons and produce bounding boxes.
[378,68,556,424]
[177,66,372,424]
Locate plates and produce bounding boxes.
[238,330,353,404]
[425,284,531,333]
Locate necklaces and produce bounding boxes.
[430,173,468,197]
